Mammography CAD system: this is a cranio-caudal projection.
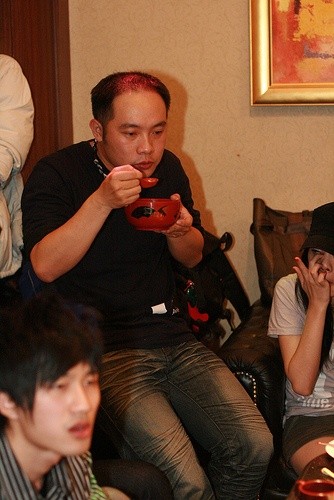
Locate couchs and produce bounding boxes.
[217,196,312,500]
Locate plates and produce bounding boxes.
[325,439,334,459]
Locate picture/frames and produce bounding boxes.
[249,0,334,107]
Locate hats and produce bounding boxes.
[305,202,334,255]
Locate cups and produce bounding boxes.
[294,479,334,500]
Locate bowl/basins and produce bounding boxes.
[124,198,181,230]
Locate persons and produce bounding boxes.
[0,53,35,307]
[267,203,334,475]
[19,72,274,500]
[0,288,174,500]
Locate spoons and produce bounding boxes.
[138,177,159,188]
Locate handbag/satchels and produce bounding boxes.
[250,195,317,308]
[179,226,232,335]
